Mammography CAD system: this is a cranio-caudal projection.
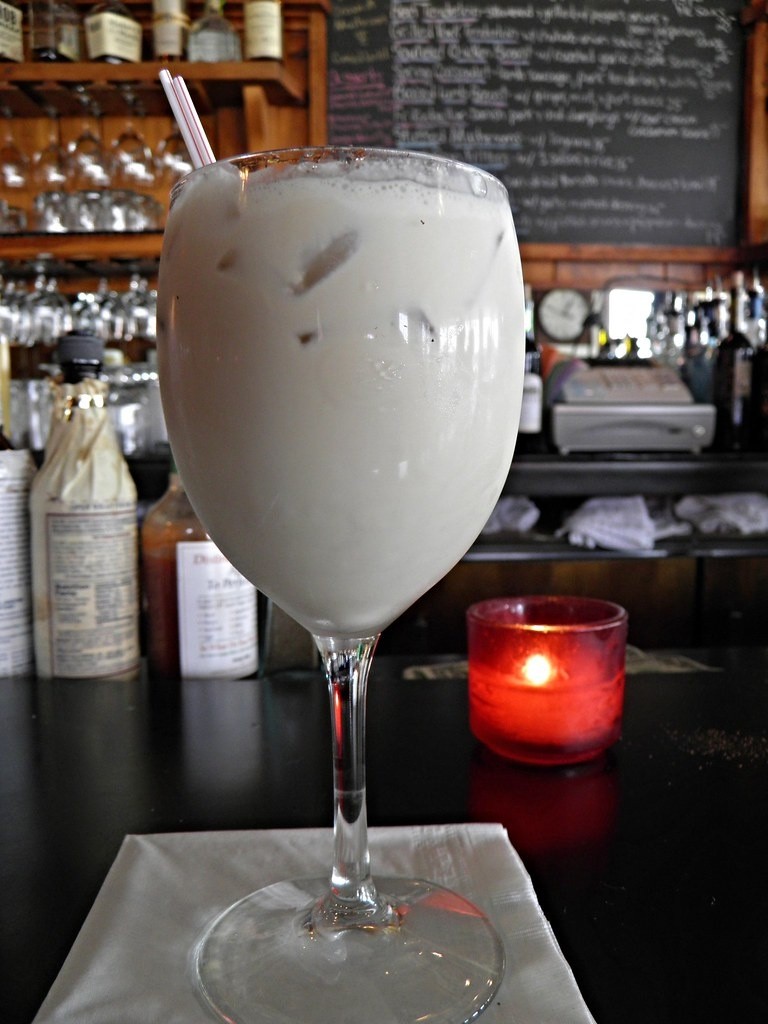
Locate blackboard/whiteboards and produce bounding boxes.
[305,1,768,263]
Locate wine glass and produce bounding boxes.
[0,259,158,345]
[0,84,195,186]
[156,147,527,1024]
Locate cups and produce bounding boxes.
[0,191,162,232]
[468,595,627,765]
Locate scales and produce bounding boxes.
[546,356,695,403]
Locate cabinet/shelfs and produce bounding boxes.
[0,0,329,380]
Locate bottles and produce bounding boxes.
[0,333,38,679]
[30,336,141,682]
[648,270,768,359]
[143,459,260,682]
[0,0,285,63]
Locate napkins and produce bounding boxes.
[34,823,596,1024]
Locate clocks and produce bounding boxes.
[538,288,591,343]
